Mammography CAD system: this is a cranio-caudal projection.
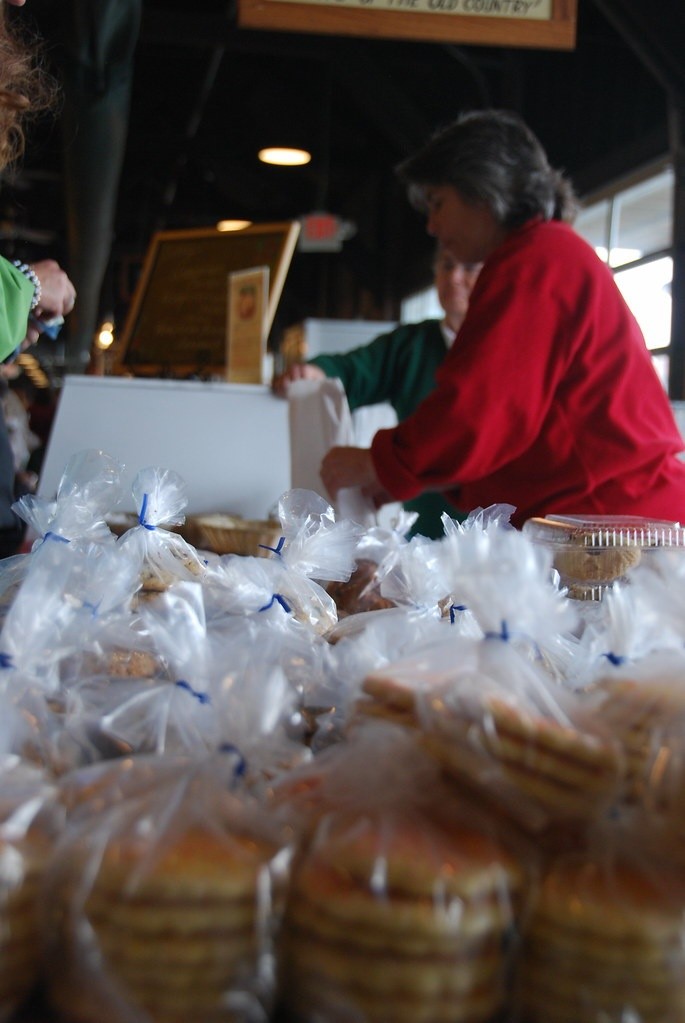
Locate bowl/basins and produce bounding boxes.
[523,513,684,605]
[192,510,283,557]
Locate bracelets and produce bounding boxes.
[13,259,42,309]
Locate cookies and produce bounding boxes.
[0,531,685,1023]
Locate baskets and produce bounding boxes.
[194,514,285,559]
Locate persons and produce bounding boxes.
[0,1,77,561]
[273,235,483,540]
[319,109,685,541]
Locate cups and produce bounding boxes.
[284,375,352,489]
[336,484,376,528]
